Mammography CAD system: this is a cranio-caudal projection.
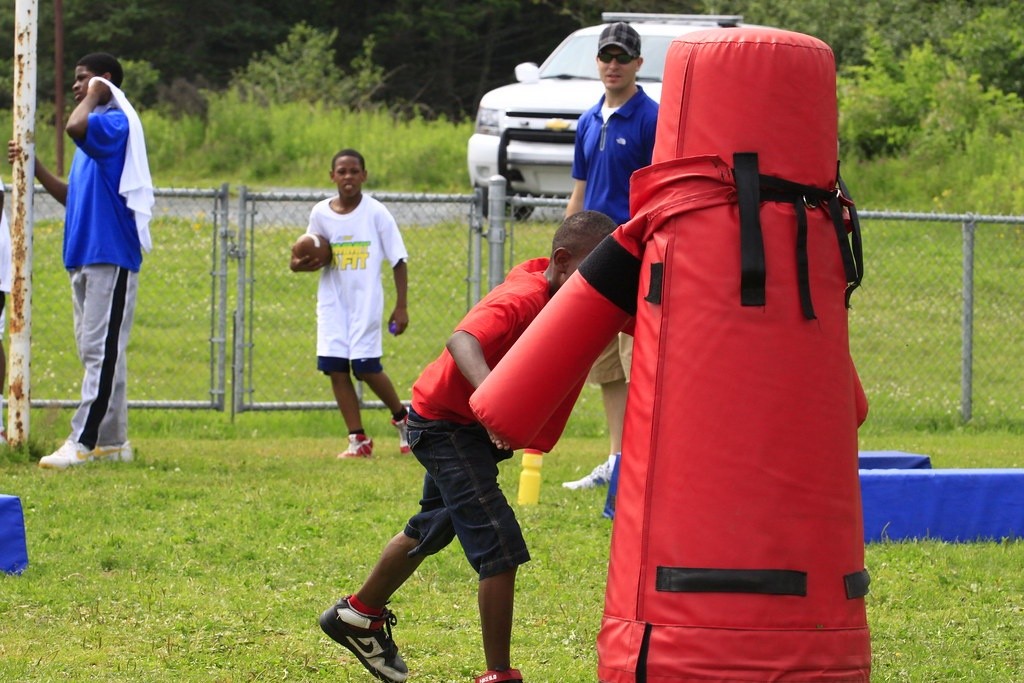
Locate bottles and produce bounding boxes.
[518,449,541,505]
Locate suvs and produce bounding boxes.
[467,3,773,221]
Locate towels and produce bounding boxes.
[87,76,156,256]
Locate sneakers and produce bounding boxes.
[94,437,133,462]
[38,441,94,471]
[320,598,408,682]
[560,462,615,492]
[339,433,373,458]
[475,669,523,683]
[391,406,411,454]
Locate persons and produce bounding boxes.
[9,53,141,472]
[558,21,662,492]
[290,148,417,460]
[318,211,624,683]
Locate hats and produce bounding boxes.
[595,22,641,59]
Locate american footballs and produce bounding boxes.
[293,232,332,267]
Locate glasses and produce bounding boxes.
[599,52,634,64]
